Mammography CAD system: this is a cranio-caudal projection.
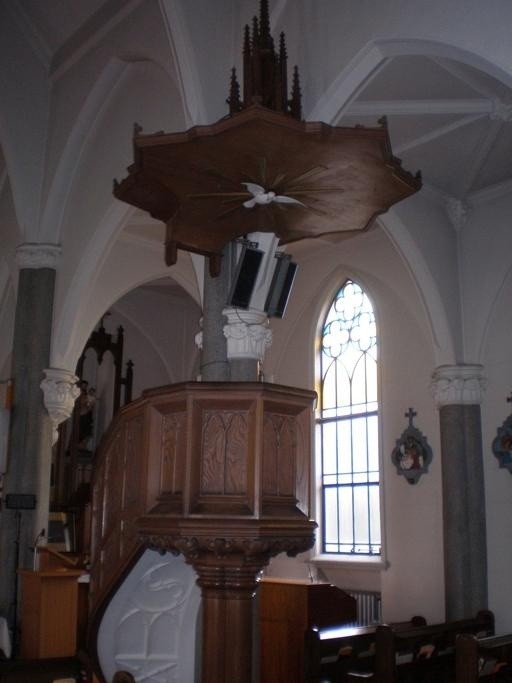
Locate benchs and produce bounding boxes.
[0,650,135,683]
[304,610,512,683]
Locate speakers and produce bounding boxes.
[264,261,299,319]
[228,245,264,311]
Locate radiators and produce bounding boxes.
[344,590,380,627]
